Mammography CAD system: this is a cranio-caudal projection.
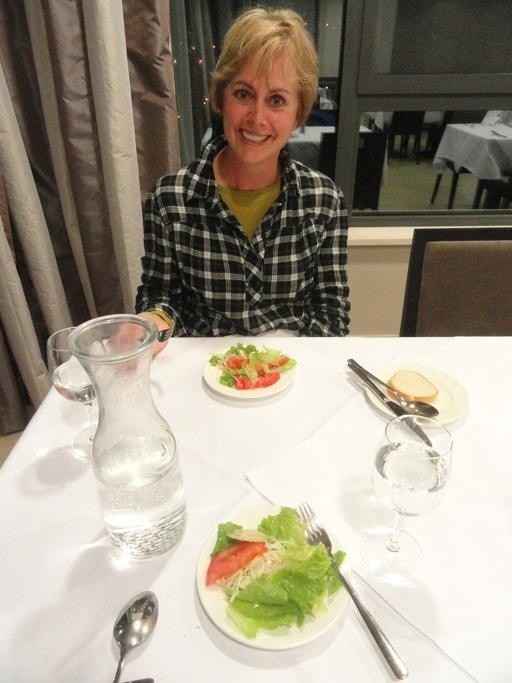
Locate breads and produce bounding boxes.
[389,368,438,407]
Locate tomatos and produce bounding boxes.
[227,355,289,390]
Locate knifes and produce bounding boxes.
[353,366,433,448]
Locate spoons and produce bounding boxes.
[107,590,161,682]
[347,356,442,417]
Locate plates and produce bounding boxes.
[194,501,344,656]
[205,347,292,399]
[367,361,470,424]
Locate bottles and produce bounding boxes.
[66,311,189,561]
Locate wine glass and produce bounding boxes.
[43,323,108,458]
[363,419,453,565]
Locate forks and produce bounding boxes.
[295,504,410,683]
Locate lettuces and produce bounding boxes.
[209,341,297,387]
[206,508,346,636]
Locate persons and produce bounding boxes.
[109,9,350,374]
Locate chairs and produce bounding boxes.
[366,110,512,159]
[431,158,482,209]
[316,130,387,210]
[471,178,511,209]
[431,110,487,155]
[387,110,423,164]
[398,225,512,336]
[304,109,337,125]
